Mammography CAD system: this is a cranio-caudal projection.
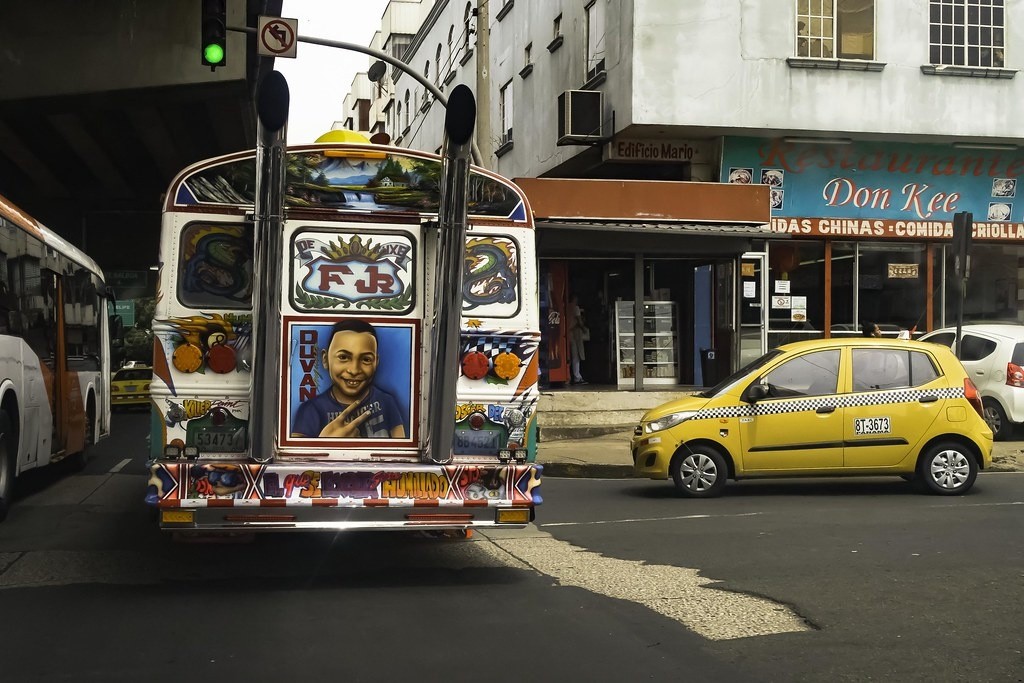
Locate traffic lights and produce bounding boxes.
[201,0,228,67]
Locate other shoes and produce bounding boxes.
[573,380,589,385]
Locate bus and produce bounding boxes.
[147,129,543,541]
[0,194,124,519]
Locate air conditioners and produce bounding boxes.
[557,89,603,140]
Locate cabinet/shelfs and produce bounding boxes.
[615,300,680,385]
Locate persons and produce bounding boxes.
[789,356,839,395]
[859,320,885,375]
[569,292,590,386]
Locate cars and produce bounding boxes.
[108,363,154,414]
[630,337,995,497]
[916,323,1024,444]
